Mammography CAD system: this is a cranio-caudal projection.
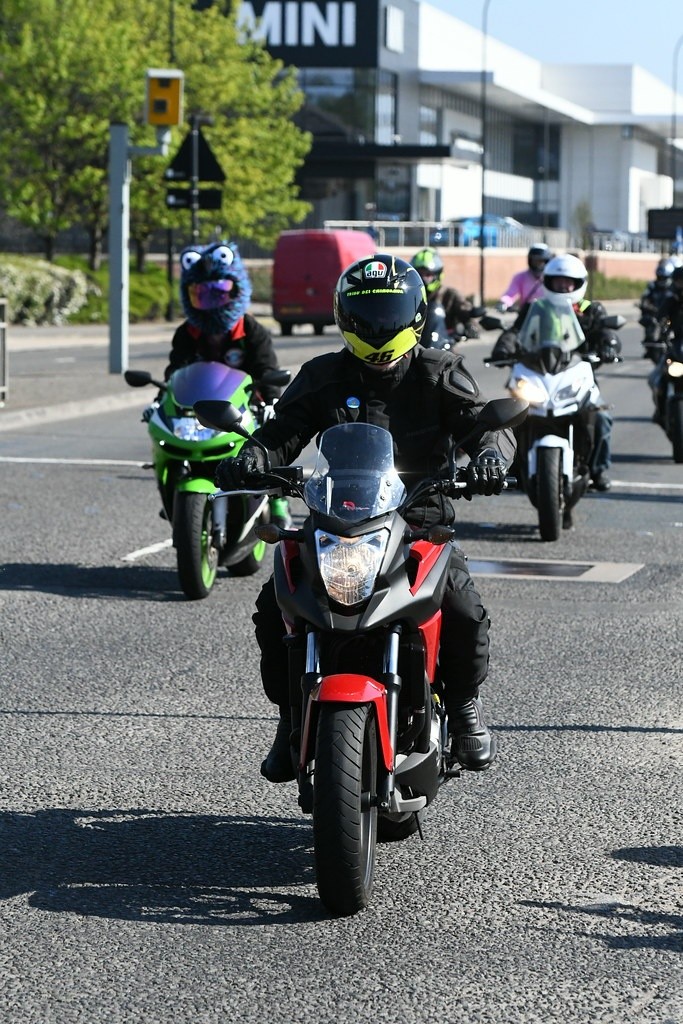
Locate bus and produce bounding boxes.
[325,219,458,246]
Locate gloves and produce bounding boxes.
[493,350,510,367]
[261,383,281,402]
[217,455,264,491]
[465,456,505,499]
[599,346,619,365]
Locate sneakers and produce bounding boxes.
[260,704,316,782]
[447,699,497,770]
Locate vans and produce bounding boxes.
[270,230,380,336]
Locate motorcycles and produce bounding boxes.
[192,401,518,916]
[123,361,290,600]
[430,295,626,542]
[636,280,683,463]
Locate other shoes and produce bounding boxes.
[594,470,611,491]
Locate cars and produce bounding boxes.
[429,217,504,247]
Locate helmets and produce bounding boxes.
[181,240,251,334]
[542,254,589,305]
[334,254,429,365]
[411,247,445,296]
[656,260,674,288]
[527,244,554,278]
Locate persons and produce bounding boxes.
[491,254,622,491]
[143,241,293,531]
[410,247,479,350]
[498,244,556,313]
[638,258,683,425]
[214,253,516,783]
